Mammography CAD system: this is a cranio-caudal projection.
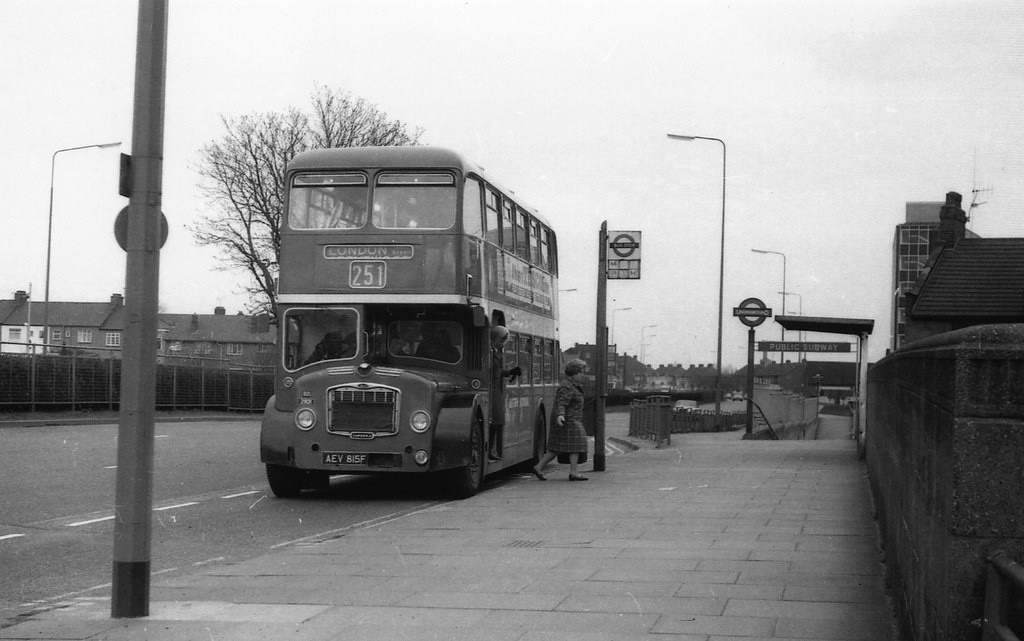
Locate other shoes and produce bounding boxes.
[569,473,589,481]
[488,454,500,460]
[533,466,547,480]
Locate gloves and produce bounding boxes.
[509,366,521,382]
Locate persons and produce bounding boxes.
[488,325,522,461]
[303,314,357,366]
[532,359,589,481]
[415,324,461,363]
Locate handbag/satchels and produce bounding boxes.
[557,451,587,464]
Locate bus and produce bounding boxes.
[259,145,562,498]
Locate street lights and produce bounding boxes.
[640,323,657,365]
[665,132,726,428]
[611,306,633,344]
[789,312,806,360]
[43,141,123,354]
[777,292,802,369]
[752,247,786,384]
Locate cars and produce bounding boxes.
[732,394,744,401]
[673,400,699,413]
